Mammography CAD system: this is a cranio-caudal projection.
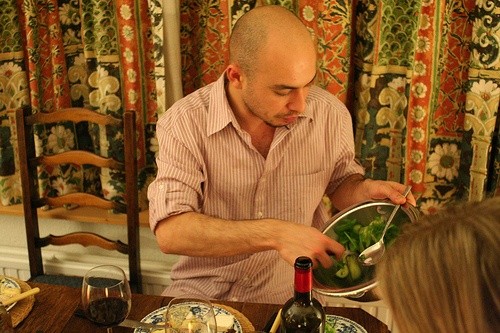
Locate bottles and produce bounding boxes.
[279,255,326,333]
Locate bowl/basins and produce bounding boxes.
[311,198,420,303]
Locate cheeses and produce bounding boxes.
[208,315,235,332]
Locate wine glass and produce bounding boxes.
[82,264,131,333]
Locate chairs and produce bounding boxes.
[14,105,142,290]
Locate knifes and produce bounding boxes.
[74,308,166,330]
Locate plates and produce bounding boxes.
[324,314,368,333]
[134,302,243,333]
[0,277,21,311]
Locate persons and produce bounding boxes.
[376,197,500,333]
[151,4,416,304]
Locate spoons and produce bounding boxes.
[358,185,413,267]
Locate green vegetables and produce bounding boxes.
[334,214,400,280]
[324,319,337,333]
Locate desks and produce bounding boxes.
[13,281,388,333]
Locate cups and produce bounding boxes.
[164,293,217,333]
